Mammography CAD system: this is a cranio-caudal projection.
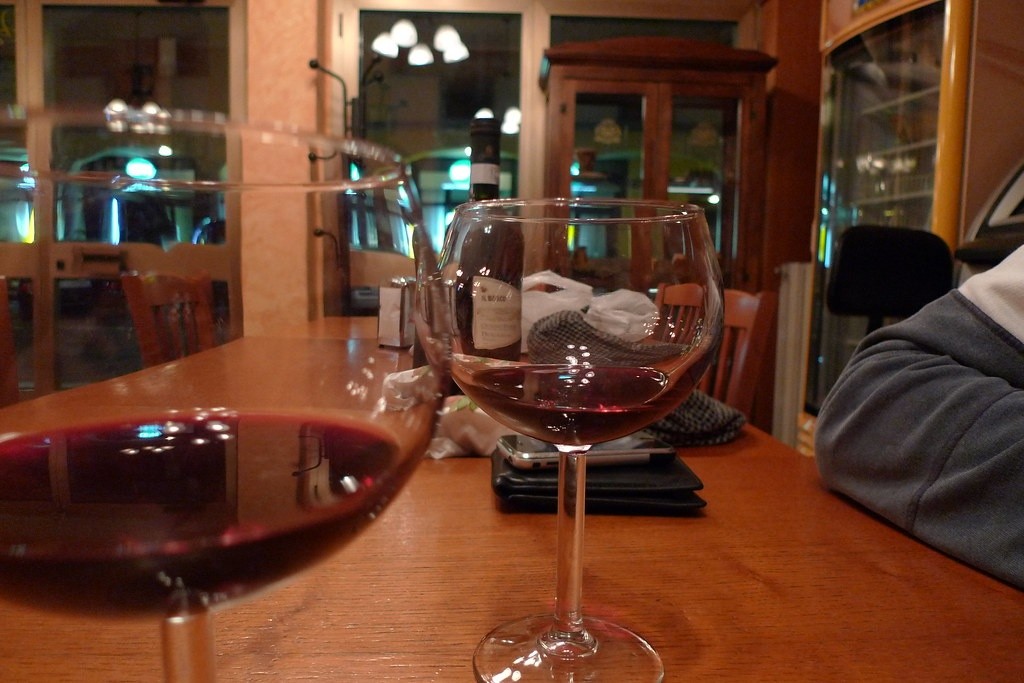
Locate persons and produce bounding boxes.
[814,243,1024,592]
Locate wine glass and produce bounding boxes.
[1,116,439,683]
[435,198,724,683]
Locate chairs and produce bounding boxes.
[120,270,218,369]
[827,226,954,335]
[640,282,779,422]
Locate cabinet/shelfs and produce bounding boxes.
[537,38,780,305]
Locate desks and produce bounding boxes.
[0,335,1024,683]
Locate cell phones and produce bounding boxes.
[498,430,678,468]
